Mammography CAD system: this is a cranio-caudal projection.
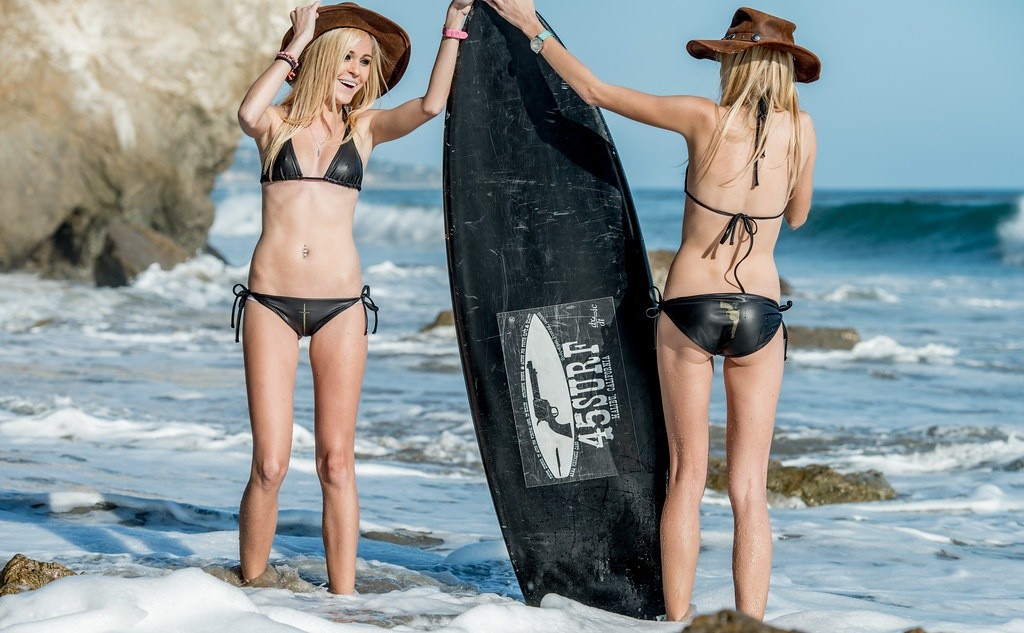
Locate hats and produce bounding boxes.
[280,3,411,105]
[686,7,821,84]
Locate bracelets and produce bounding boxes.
[443,28,468,40]
[274,51,299,80]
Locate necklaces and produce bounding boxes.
[307,123,334,159]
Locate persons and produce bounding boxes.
[484,0,821,622]
[238,0,471,596]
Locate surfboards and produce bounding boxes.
[443,3,670,618]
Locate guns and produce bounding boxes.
[524,359,573,442]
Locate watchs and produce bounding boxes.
[529,29,553,54]
